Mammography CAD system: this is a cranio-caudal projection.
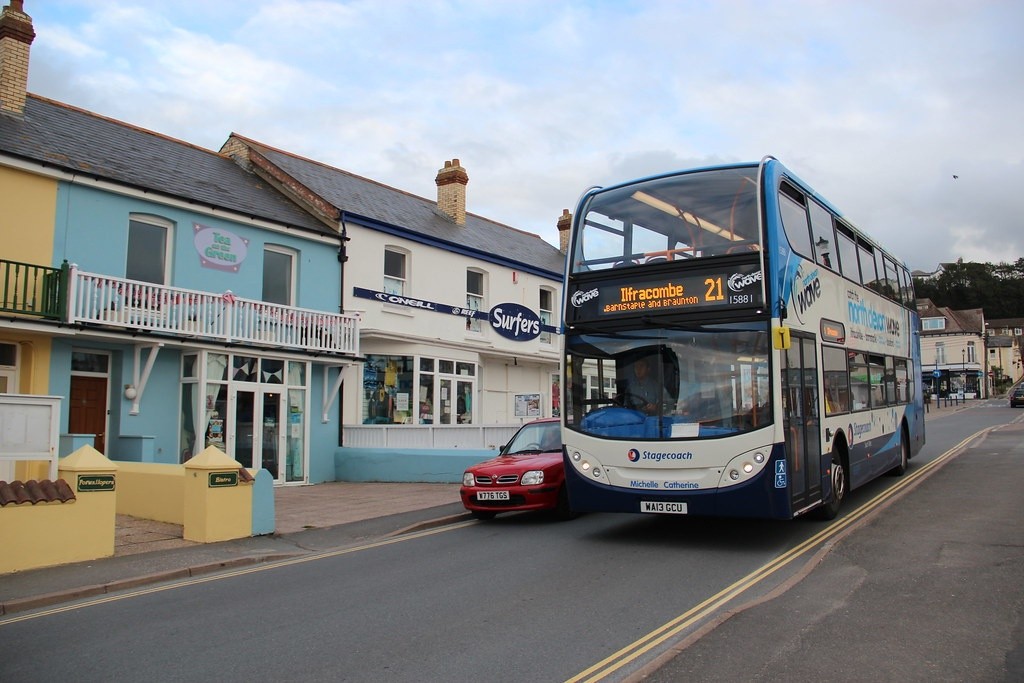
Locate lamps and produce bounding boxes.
[124,383,138,399]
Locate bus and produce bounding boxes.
[559,154,926,520]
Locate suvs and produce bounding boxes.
[1011,388,1024,407]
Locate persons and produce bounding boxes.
[824,377,844,413]
[622,357,674,415]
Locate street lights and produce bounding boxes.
[961,349,966,403]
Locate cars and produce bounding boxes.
[460,417,573,522]
